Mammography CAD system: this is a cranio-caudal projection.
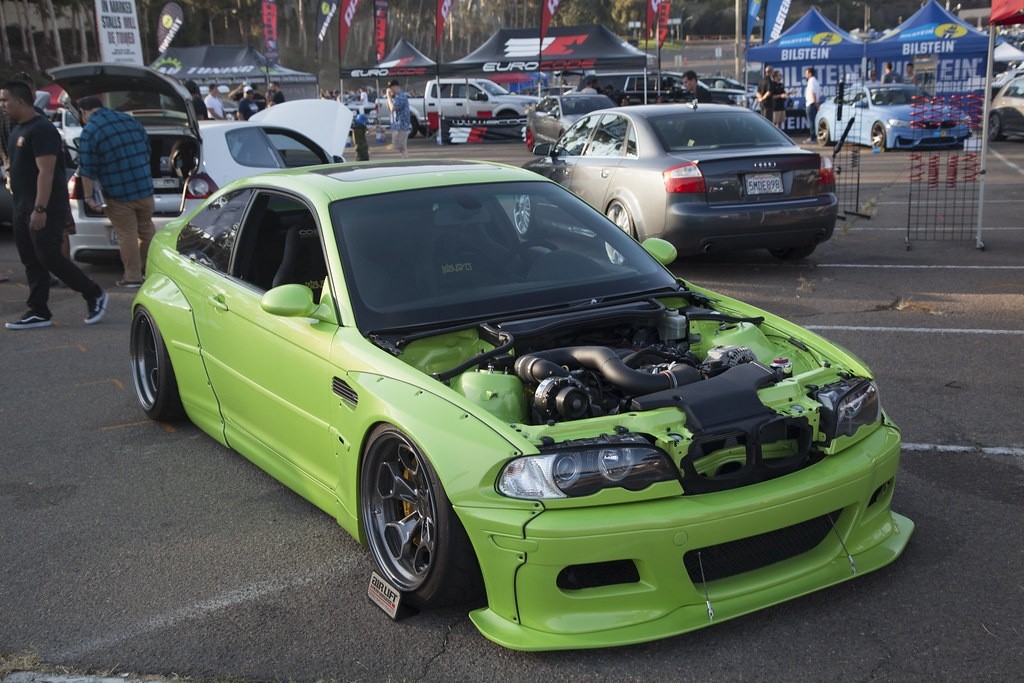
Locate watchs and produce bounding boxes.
[34,205,46,213]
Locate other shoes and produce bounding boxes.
[803,137,816,144]
[116,280,142,287]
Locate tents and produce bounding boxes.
[975,0,1024,250]
[340,24,658,146]
[146,44,319,98]
[744,0,990,136]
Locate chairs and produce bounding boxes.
[270,217,327,304]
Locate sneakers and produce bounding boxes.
[5,313,52,329]
[84,291,109,323]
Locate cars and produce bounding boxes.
[1,62,354,261]
[989,68,1024,141]
[615,71,757,107]
[523,93,616,152]
[521,99,840,262]
[129,157,913,653]
[815,83,974,152]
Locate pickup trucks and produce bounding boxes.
[376,78,542,139]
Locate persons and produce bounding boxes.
[385,79,411,149]
[237,85,259,121]
[772,70,789,128]
[881,62,901,83]
[183,80,207,120]
[77,96,156,288]
[756,67,774,121]
[865,70,880,84]
[580,81,597,94]
[229,82,267,111]
[265,82,285,107]
[681,70,711,103]
[320,89,376,103]
[0,72,109,330]
[803,67,821,144]
[903,63,915,84]
[204,84,227,119]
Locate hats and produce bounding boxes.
[387,80,398,88]
[244,86,253,92]
